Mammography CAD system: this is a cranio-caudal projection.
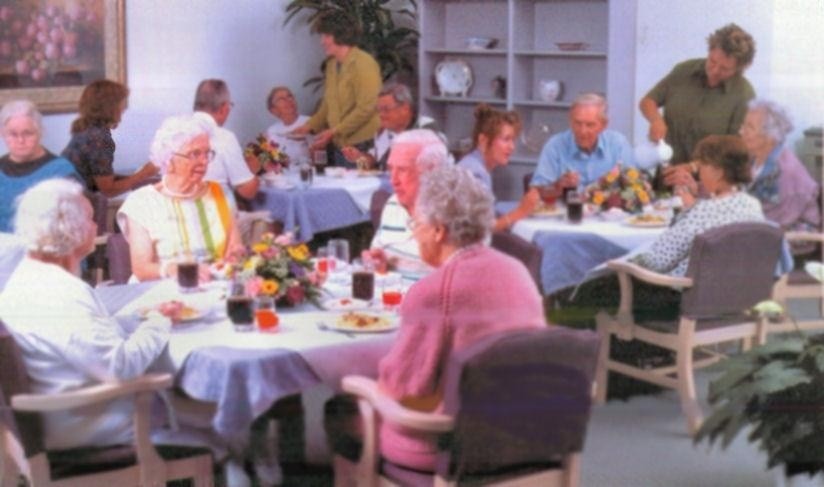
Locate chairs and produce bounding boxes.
[1,320,214,486]
[332,325,602,486]
[596,221,783,436]
[742,187,822,344]
[0,171,559,289]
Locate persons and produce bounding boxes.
[530,92,639,202]
[591,133,767,403]
[342,83,448,171]
[114,112,282,486]
[321,165,549,487]
[639,23,756,190]
[149,79,273,252]
[458,106,541,233]
[669,96,822,274]
[264,86,327,170]
[361,128,492,281]
[1,103,88,278]
[60,81,161,268]
[286,10,384,169]
[2,178,281,487]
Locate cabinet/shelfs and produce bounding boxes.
[414,0,636,204]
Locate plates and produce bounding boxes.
[555,42,585,51]
[435,56,475,98]
[531,182,682,228]
[460,36,499,48]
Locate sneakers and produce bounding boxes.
[247,436,284,487]
[607,368,664,402]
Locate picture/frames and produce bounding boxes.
[0,0,129,115]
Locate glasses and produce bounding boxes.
[169,148,215,164]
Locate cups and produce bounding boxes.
[262,166,370,188]
[143,238,406,333]
[313,150,327,173]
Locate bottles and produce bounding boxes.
[538,78,559,101]
[489,74,508,100]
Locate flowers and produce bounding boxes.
[241,131,292,175]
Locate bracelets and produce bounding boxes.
[159,262,170,279]
[690,162,699,174]
[504,217,513,226]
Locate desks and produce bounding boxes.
[489,202,692,353]
[104,267,418,486]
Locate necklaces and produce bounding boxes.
[162,175,197,197]
[447,244,480,266]
